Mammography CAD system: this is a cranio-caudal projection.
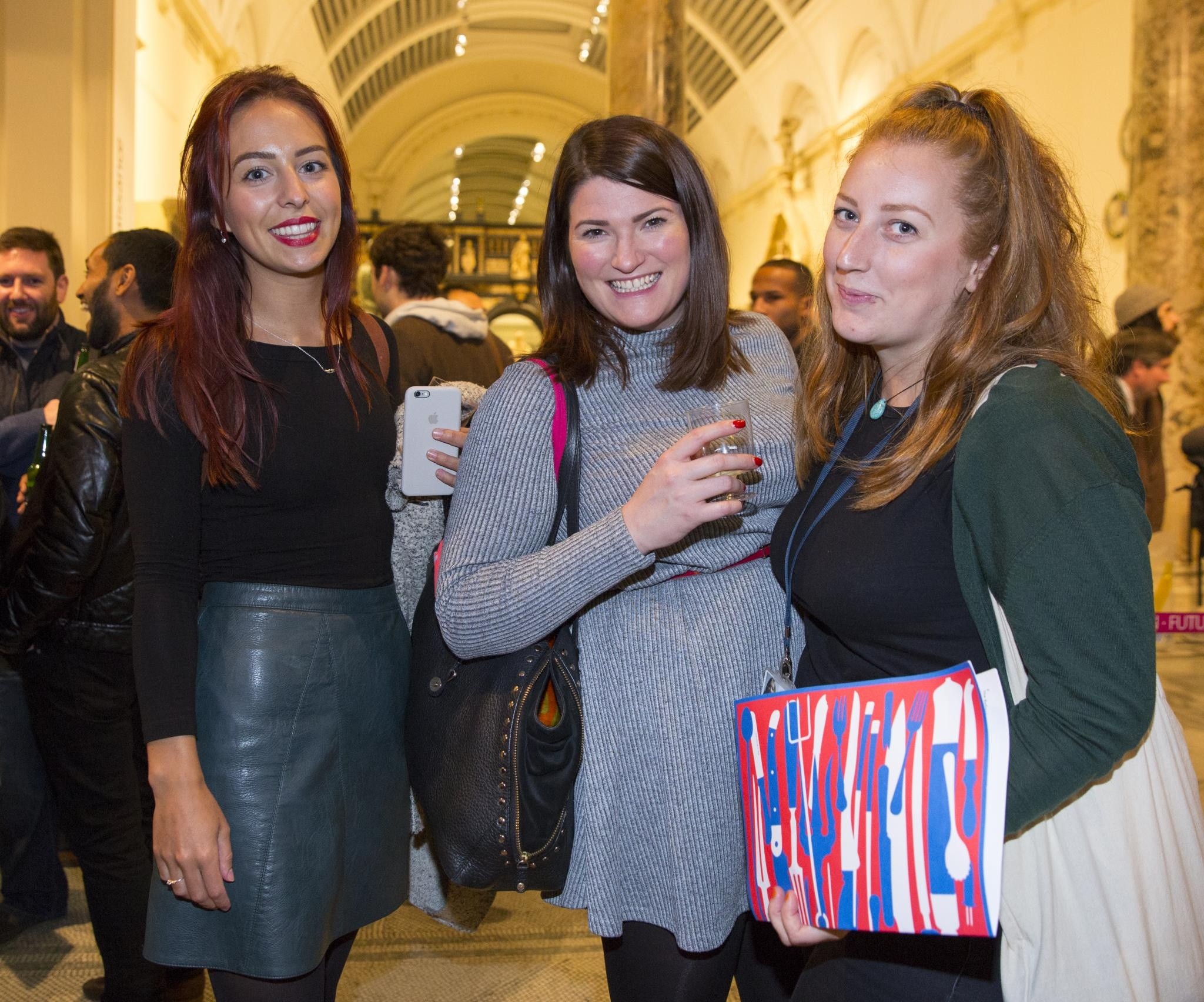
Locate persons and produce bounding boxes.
[434,116,803,1002]
[439,284,486,318]
[78,64,471,1002]
[1081,287,1183,542]
[0,226,205,1002]
[367,223,515,398]
[761,678,776,695]
[727,84,1204,1002]
[747,259,817,383]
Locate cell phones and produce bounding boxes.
[402,386,462,495]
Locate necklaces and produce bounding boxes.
[241,309,339,374]
[870,375,927,420]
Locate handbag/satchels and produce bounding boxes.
[409,361,582,892]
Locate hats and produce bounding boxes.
[1115,282,1169,328]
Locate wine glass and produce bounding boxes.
[684,398,758,505]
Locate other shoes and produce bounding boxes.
[83,976,105,1002]
[56,838,80,866]
[0,887,69,948]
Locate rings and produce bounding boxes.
[166,877,184,885]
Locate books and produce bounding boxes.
[733,661,1009,937]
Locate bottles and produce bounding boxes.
[73,342,91,373]
[24,423,52,505]
